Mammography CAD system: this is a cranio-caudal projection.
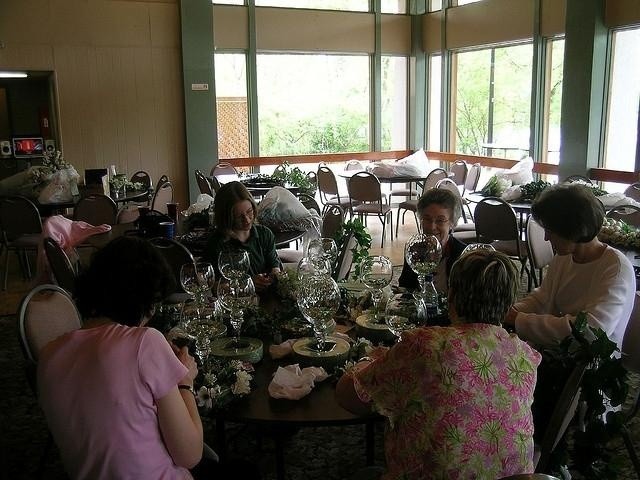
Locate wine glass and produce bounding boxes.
[157,236,499,394]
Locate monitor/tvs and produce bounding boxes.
[12,137,44,157]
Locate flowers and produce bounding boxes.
[41,147,71,171]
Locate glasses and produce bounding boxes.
[420,217,450,225]
[235,210,253,217]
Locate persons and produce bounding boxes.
[36,232,222,480]
[334,248,543,479]
[200,180,287,298]
[502,182,637,477]
[14,139,27,156]
[397,188,469,297]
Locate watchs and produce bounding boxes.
[357,355,374,363]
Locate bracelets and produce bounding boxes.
[176,382,199,398]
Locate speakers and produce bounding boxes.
[44,140,55,154]
[0,141,11,155]
